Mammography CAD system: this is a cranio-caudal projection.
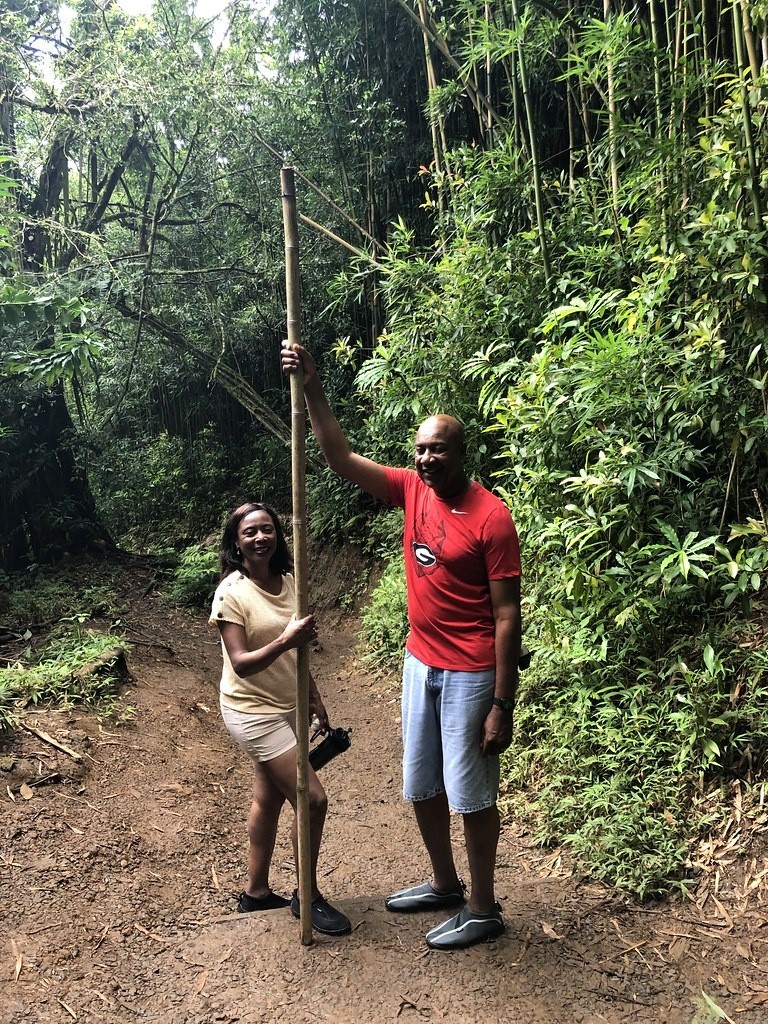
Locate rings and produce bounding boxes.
[312,628,315,631]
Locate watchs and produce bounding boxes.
[494,697,515,712]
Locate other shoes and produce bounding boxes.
[425,895,509,950]
[384,880,468,912]
[290,888,351,935]
[236,891,291,913]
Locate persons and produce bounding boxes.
[205,503,358,937]
[280,340,522,949]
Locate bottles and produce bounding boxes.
[305,726,351,772]
[311,713,321,731]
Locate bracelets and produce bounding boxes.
[309,691,320,700]
